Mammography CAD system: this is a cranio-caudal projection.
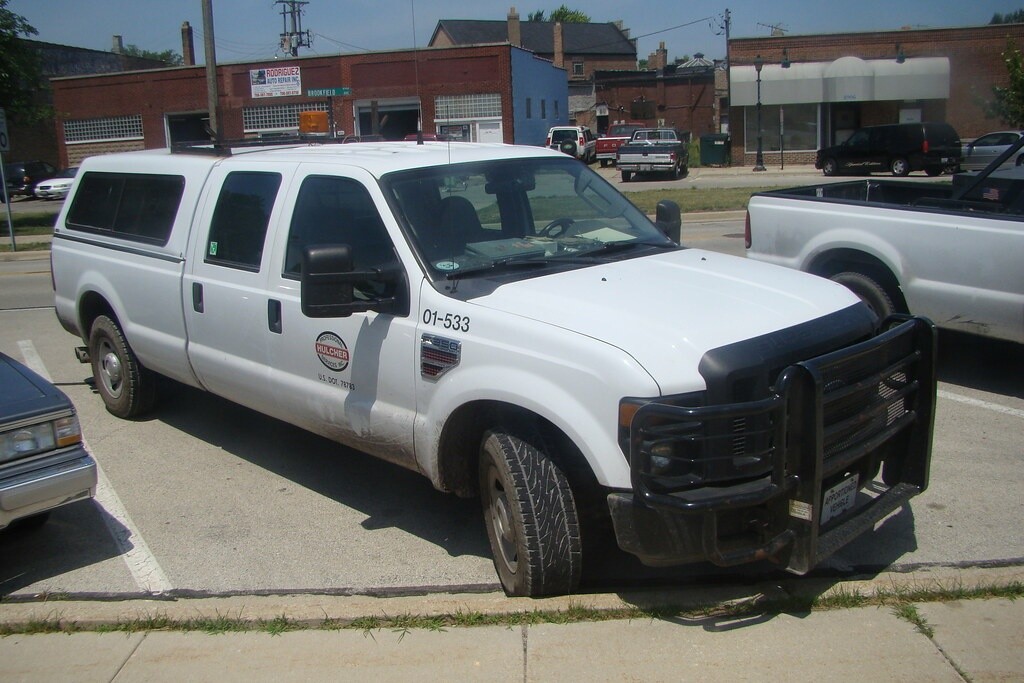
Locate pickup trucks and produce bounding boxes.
[745,129,1024,351]
[598,123,689,181]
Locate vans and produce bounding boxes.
[45,125,940,603]
[813,123,960,178]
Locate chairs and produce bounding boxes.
[424,194,489,256]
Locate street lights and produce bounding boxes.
[751,54,767,171]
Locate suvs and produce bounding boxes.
[544,124,596,165]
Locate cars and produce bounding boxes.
[0,158,59,204]
[0,350,96,530]
[35,165,80,200]
